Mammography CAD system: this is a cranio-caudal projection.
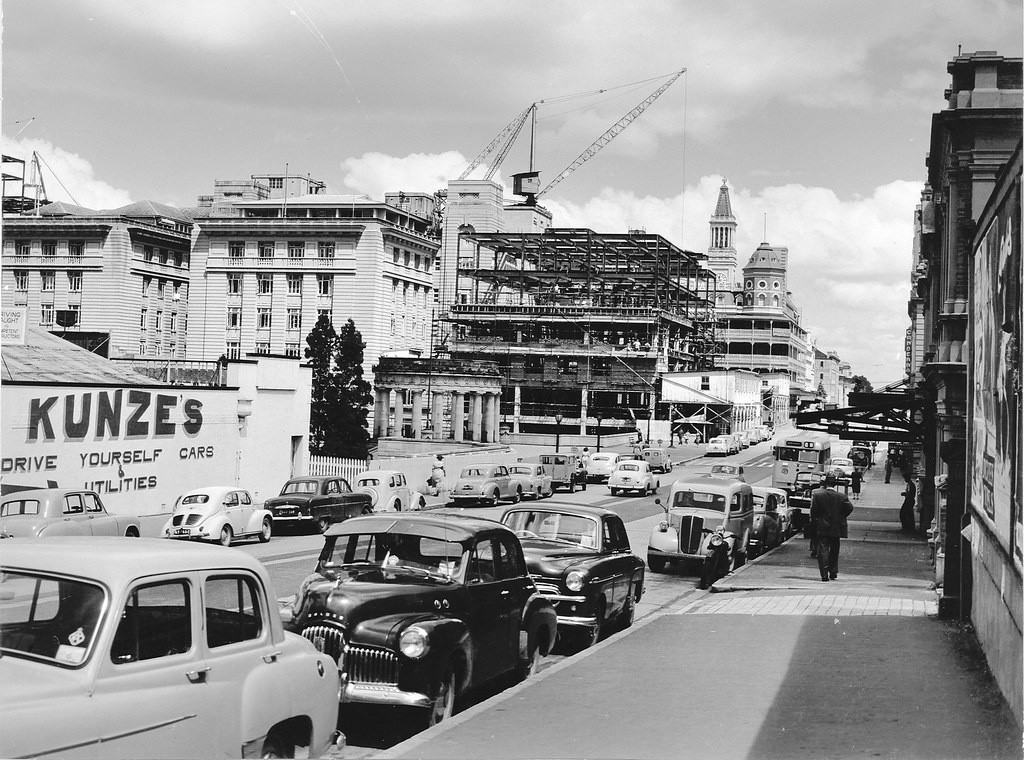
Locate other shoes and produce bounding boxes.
[829,573,838,579]
[821,576,829,581]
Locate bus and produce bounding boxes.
[772,436,831,492]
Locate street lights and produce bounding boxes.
[555,409,563,453]
[646,408,676,449]
[597,410,602,452]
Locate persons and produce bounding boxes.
[899,472,916,533]
[550,283,565,294]
[851,466,867,500]
[633,426,650,460]
[582,448,591,468]
[884,454,893,485]
[809,470,853,582]
[626,339,650,351]
[430,454,446,496]
[681,493,696,507]
[676,427,701,445]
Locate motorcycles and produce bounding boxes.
[700,525,743,588]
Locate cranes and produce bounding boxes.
[432,67,688,250]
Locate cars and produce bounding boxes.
[263,476,372,533]
[705,421,776,457]
[507,453,588,499]
[787,471,832,509]
[448,464,525,507]
[0,536,346,759]
[277,511,557,730]
[0,489,141,540]
[829,441,877,486]
[473,501,647,649]
[353,471,426,512]
[587,448,672,496]
[647,464,795,571]
[159,487,274,546]
